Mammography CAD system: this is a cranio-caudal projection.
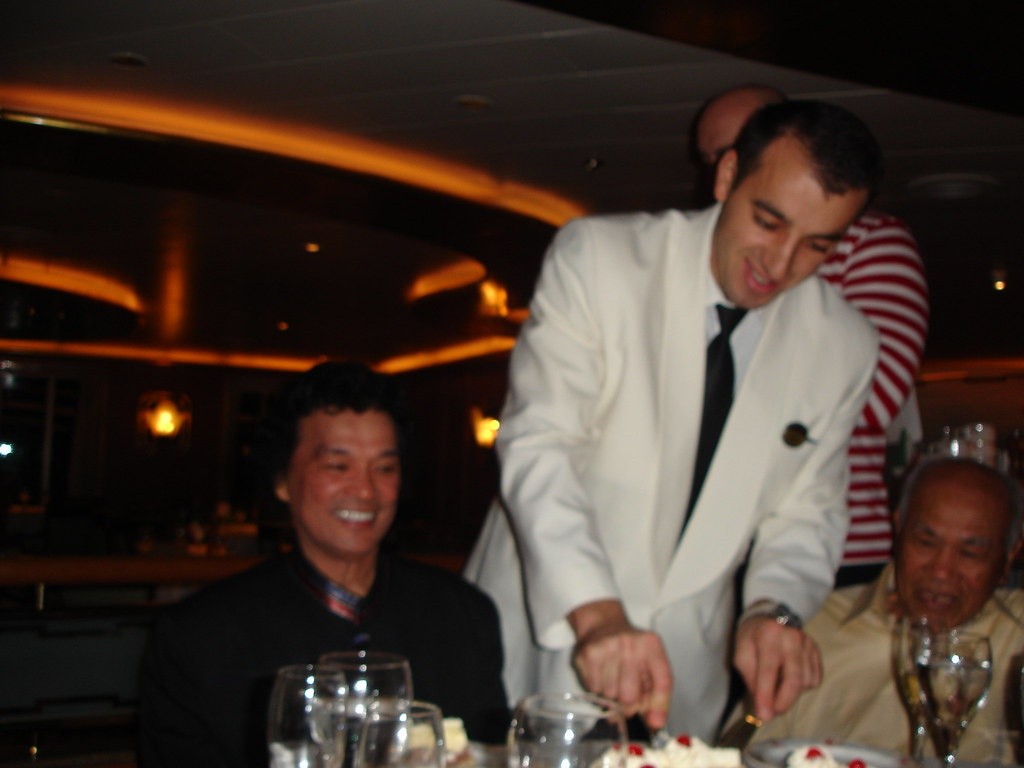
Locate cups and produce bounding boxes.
[505,690,631,768]
[355,697,448,768]
[319,652,413,768]
[268,664,350,768]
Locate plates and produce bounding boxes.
[744,735,908,768]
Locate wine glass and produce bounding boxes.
[890,613,949,768]
[910,631,995,768]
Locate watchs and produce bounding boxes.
[739,602,802,629]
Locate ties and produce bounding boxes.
[677,303,749,540]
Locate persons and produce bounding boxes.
[720,457,1024,768]
[696,91,930,590]
[137,360,508,767]
[462,98,884,749]
